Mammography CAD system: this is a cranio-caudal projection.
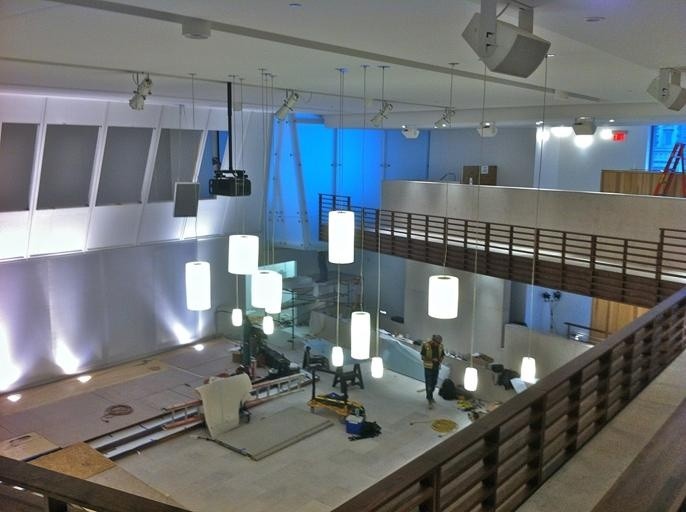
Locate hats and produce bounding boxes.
[431,334,443,344]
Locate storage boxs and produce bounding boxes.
[345,414,364,435]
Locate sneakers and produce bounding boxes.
[425,395,436,410]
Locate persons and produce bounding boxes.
[420,334,446,410]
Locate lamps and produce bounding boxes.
[331,66,345,368]
[351,62,372,362]
[328,66,356,266]
[232,77,242,328]
[542,291,551,300]
[133,77,153,109]
[186,74,209,312]
[553,291,562,301]
[371,66,390,378]
[477,80,497,138]
[259,72,273,336]
[371,102,393,126]
[461,13,551,79]
[647,66,686,111]
[428,62,460,321]
[573,115,595,137]
[435,111,456,128]
[274,90,299,121]
[464,61,488,392]
[227,71,259,275]
[520,54,550,382]
[401,125,419,140]
[251,69,282,308]
[268,71,281,315]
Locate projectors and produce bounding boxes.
[208,169,251,196]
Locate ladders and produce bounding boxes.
[161,373,306,429]
[652,142,686,198]
